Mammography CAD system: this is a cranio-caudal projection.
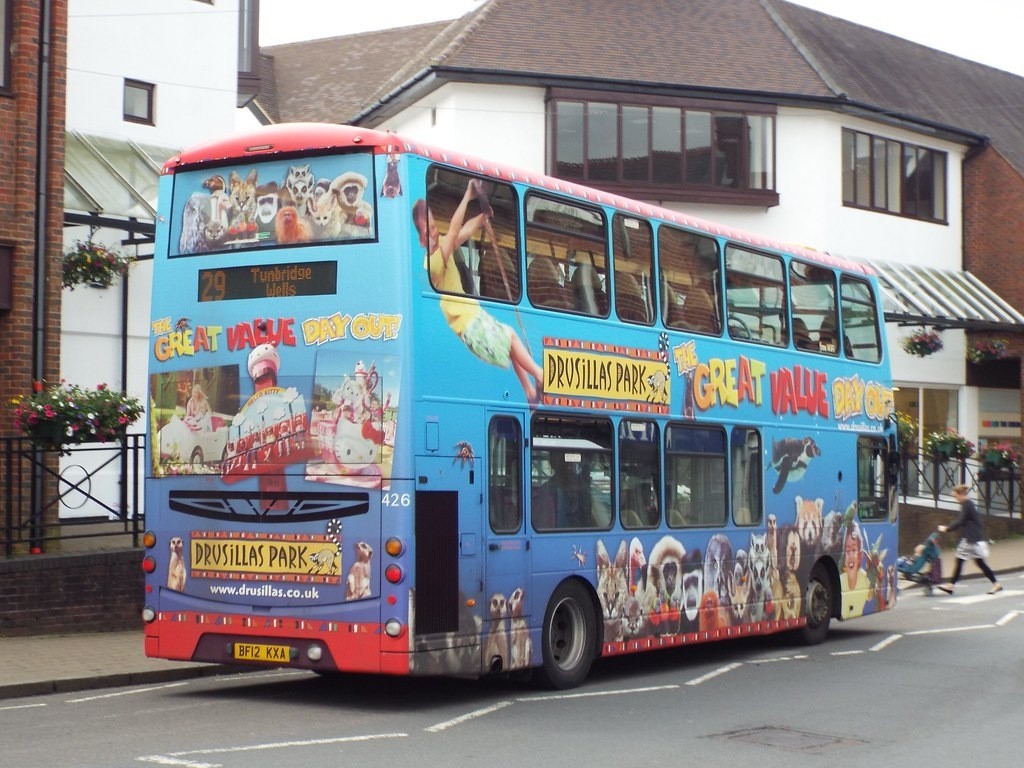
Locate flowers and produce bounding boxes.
[978,442,1022,469]
[896,412,927,449]
[924,426,976,462]
[59,240,133,290]
[966,334,1010,365]
[899,327,944,357]
[8,378,144,445]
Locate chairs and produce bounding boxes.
[784,315,853,358]
[623,509,687,528]
[453,245,722,336]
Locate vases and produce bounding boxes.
[985,450,1003,467]
[937,442,954,456]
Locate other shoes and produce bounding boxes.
[938,585,952,596]
[988,586,1004,595]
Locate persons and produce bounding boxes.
[898,543,926,574]
[412,178,544,413]
[934,484,1004,595]
[841,523,874,619]
[547,447,592,529]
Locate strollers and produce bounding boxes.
[897,527,944,598]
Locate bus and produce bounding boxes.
[141,122,901,691]
